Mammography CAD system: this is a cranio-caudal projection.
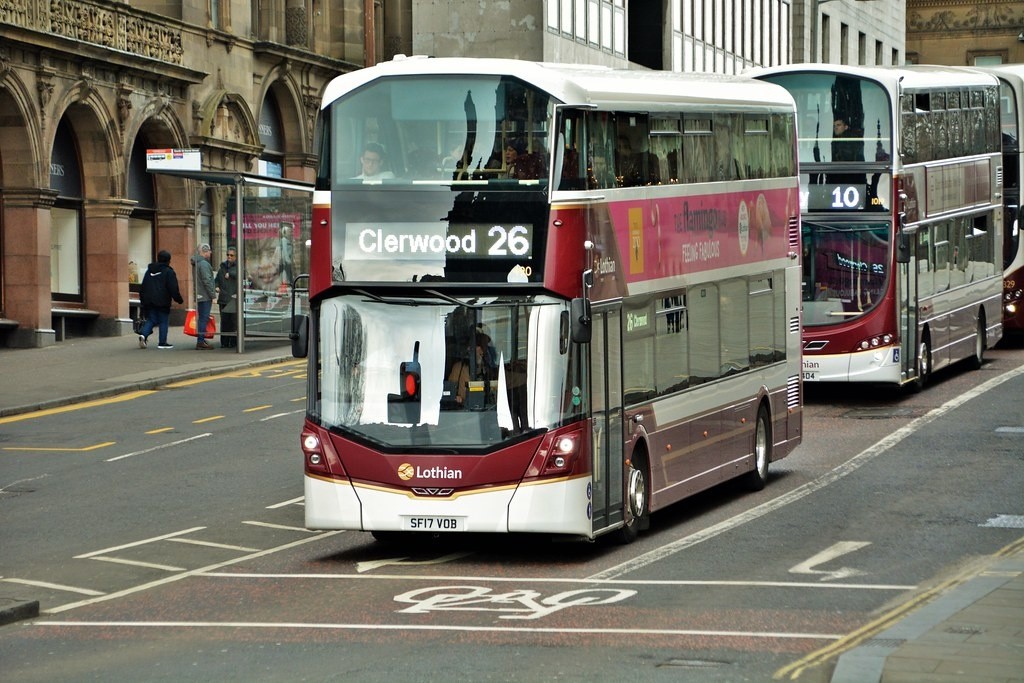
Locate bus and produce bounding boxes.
[742,62,1024,395]
[290,54,805,552]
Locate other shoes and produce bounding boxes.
[158,343,174,349]
[138,335,147,348]
[196,341,213,350]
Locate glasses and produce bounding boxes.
[227,254,235,256]
[362,158,381,164]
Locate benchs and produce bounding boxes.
[51,301,101,343]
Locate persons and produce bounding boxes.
[592,136,642,190]
[214,247,237,348]
[191,243,217,350]
[137,250,183,348]
[434,138,528,179]
[350,143,395,179]
[448,346,483,403]
[281,226,294,286]
[244,237,283,289]
[813,117,864,161]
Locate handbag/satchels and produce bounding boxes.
[133,316,152,334]
[184,309,216,338]
